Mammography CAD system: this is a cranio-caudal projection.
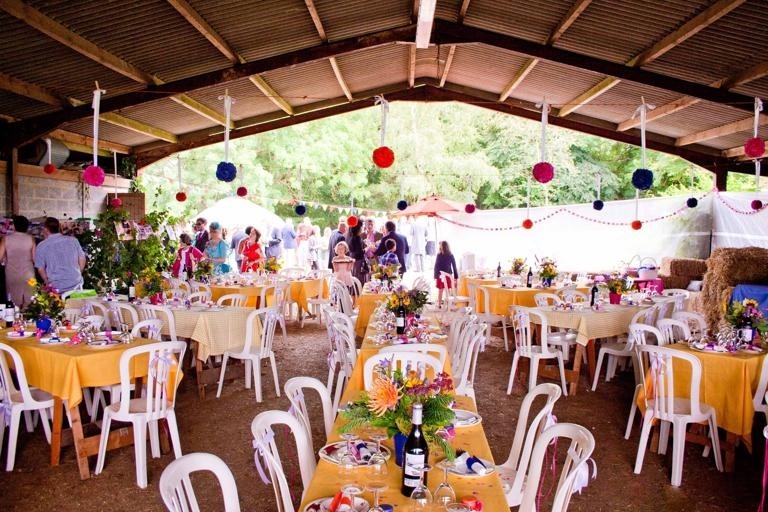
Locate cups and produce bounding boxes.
[202,272,278,285]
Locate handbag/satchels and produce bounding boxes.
[222,264,232,273]
[192,260,198,272]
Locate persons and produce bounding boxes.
[33,217,86,300]
[433,241,458,307]
[410,218,428,273]
[171,214,410,312]
[0,215,37,312]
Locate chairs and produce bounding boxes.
[414,265,767,512]
[1,265,363,512]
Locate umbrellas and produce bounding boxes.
[400,192,460,245]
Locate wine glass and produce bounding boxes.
[339,431,468,512]
[120,323,133,344]
[552,295,587,311]
[687,323,738,354]
[621,284,657,305]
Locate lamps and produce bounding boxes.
[415,0,436,49]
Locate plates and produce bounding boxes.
[439,457,496,479]
[447,409,482,428]
[363,280,387,293]
[162,297,221,313]
[738,344,761,353]
[368,309,441,346]
[302,495,368,512]
[6,328,125,350]
[316,440,391,468]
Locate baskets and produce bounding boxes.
[638,257,657,280]
[627,255,641,277]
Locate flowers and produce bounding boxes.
[533,254,558,277]
[509,258,528,274]
[363,239,380,265]
[257,256,287,272]
[193,258,216,284]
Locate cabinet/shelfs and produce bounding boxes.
[107,192,145,221]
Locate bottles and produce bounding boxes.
[127,275,136,303]
[4,293,15,328]
[741,309,753,347]
[496,261,501,277]
[400,401,429,499]
[383,269,388,282]
[395,296,406,335]
[525,266,532,288]
[590,281,598,307]
